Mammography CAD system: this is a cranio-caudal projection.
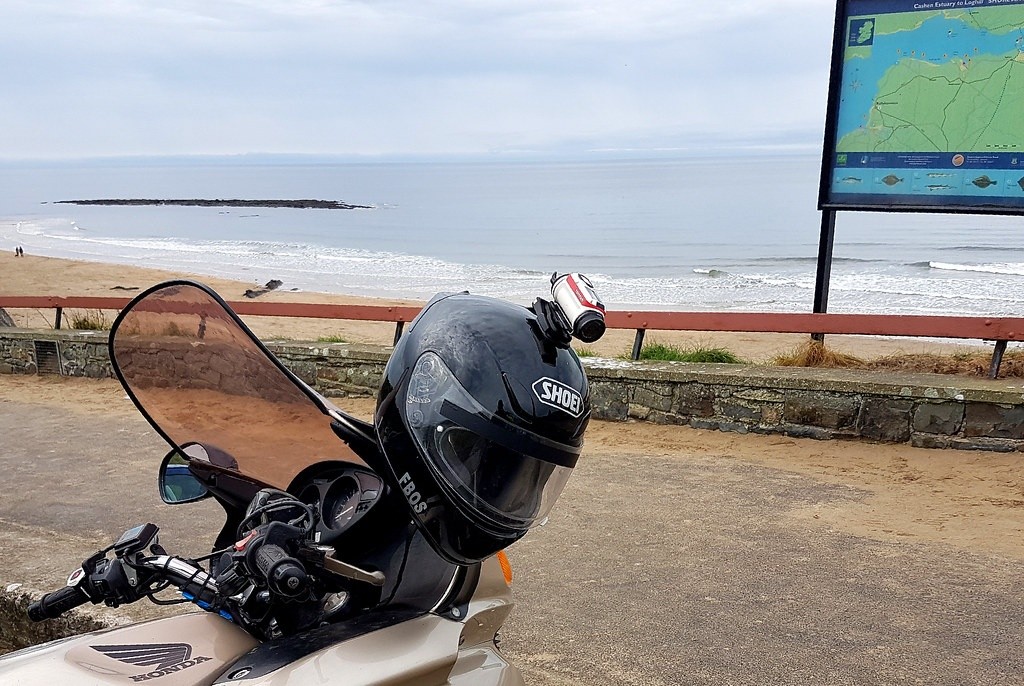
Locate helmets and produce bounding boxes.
[372,291,593,567]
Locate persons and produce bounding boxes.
[16,246,23,257]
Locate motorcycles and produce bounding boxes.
[0,269,607,685]
